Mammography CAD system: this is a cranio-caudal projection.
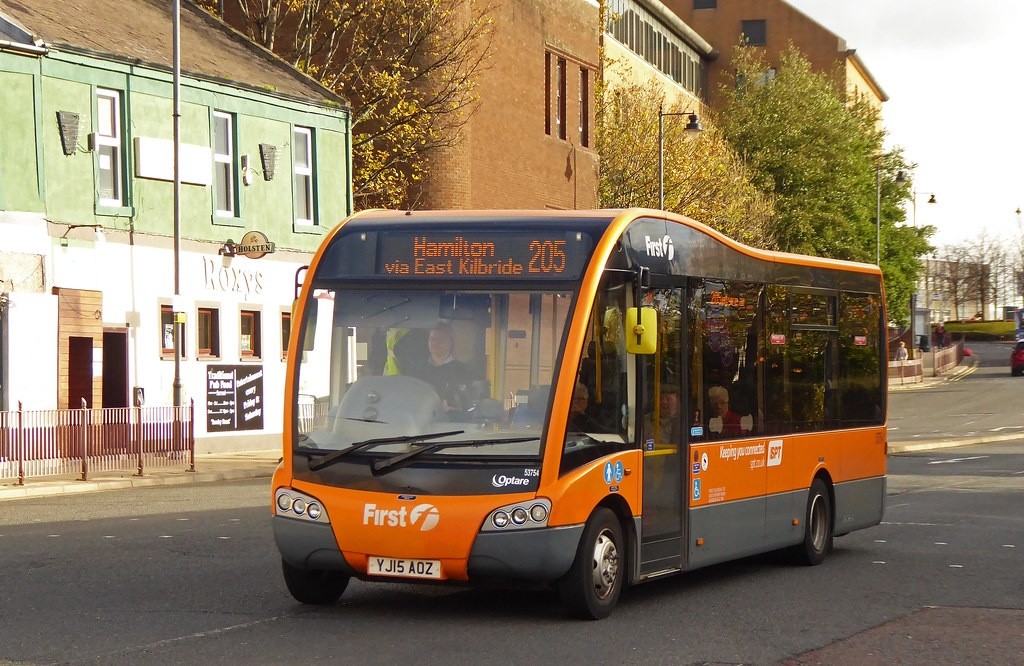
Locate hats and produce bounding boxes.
[428,322,456,345]
[660,384,677,394]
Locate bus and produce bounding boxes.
[271,207,888,624]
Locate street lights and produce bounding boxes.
[876,161,909,267]
[659,97,705,210]
[909,190,939,359]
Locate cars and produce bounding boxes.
[1011,339,1024,377]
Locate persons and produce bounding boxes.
[415,321,469,417]
[568,381,755,445]
